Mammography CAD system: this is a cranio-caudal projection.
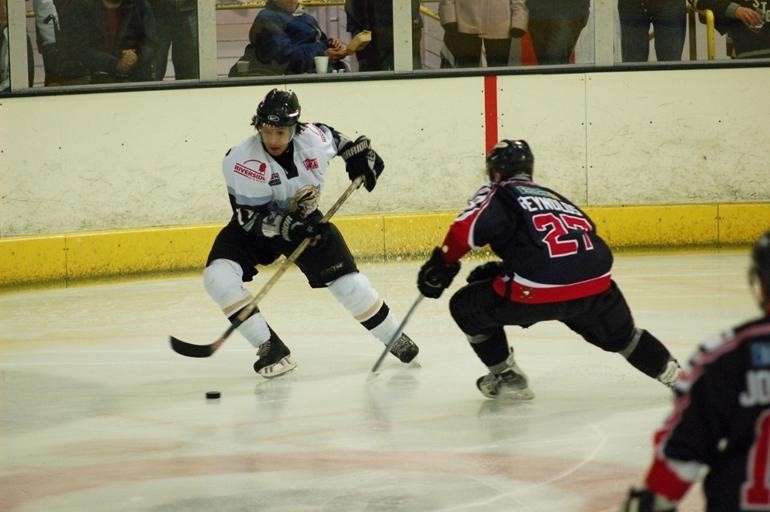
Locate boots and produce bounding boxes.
[473,348,529,399]
[384,332,419,364]
[252,321,291,372]
[660,358,694,403]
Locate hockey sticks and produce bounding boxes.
[370,273,437,373]
[173,174,364,356]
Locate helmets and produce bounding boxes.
[750,231,770,308]
[253,86,301,129]
[484,139,535,184]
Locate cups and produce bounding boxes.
[314,55,329,75]
[236,61,249,74]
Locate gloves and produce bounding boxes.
[279,210,330,250]
[415,244,462,300]
[338,135,385,192]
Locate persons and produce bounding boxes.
[417,139,693,400]
[620,229,770,511]
[200,86,418,382]
[2,1,768,93]
